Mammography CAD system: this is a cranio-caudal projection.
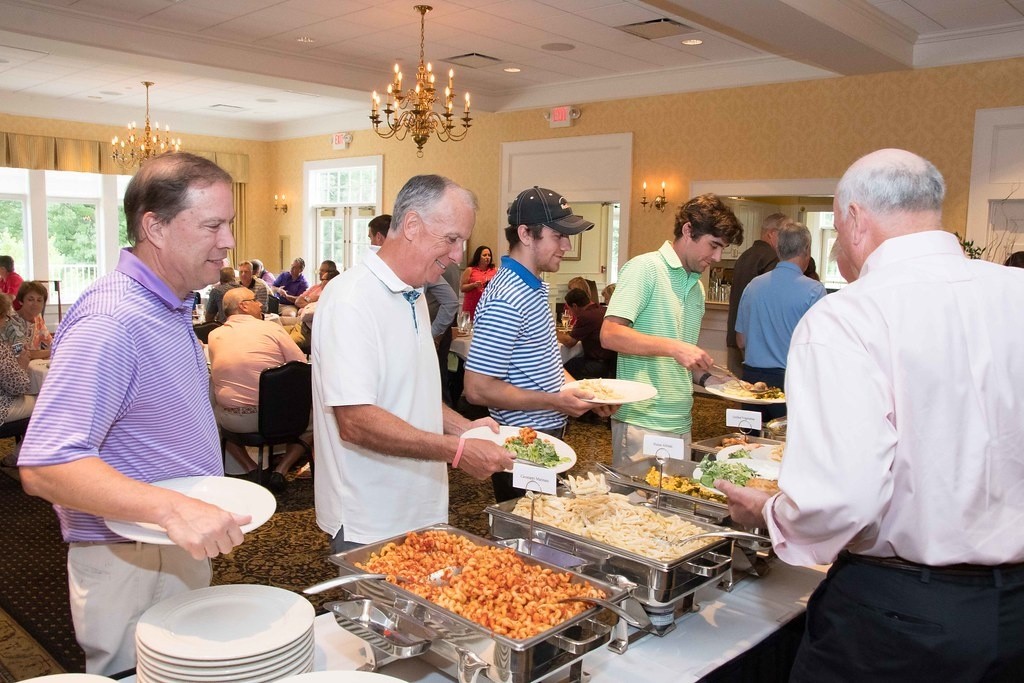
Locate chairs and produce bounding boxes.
[220,360,314,486]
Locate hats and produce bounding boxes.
[508,185,595,235]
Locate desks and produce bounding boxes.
[449,327,584,401]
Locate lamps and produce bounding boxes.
[111,81,181,170]
[640,182,668,213]
[274,194,288,213]
[369,5,472,158]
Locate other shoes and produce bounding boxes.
[270,471,287,489]
[250,469,268,481]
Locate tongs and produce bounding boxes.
[589,458,650,486]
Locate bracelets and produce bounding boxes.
[452,438,466,468]
[699,373,712,388]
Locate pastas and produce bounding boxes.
[355,531,608,641]
[510,471,724,562]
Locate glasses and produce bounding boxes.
[242,297,258,303]
[5,314,11,323]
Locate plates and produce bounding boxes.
[705,384,786,405]
[560,379,658,405]
[461,425,577,473]
[104,476,277,546]
[12,584,410,683]
[694,458,782,496]
[716,443,784,461]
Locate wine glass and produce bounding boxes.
[561,310,573,332]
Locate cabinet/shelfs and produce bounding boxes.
[713,197,794,260]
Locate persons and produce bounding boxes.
[726,212,792,381]
[803,257,820,282]
[442,264,461,327]
[368,214,459,407]
[735,222,827,436]
[713,148,1024,683]
[460,246,498,320]
[463,180,622,503]
[0,255,53,426]
[208,287,312,492]
[829,237,859,283]
[599,192,744,466]
[556,276,616,380]
[311,173,516,555]
[18,153,251,676]
[201,257,340,355]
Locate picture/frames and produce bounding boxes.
[560,216,582,261]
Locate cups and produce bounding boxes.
[457,310,471,337]
[708,277,731,300]
[196,304,204,315]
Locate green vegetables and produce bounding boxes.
[502,438,571,467]
[696,446,759,490]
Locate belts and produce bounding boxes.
[843,552,1024,575]
[224,406,258,414]
